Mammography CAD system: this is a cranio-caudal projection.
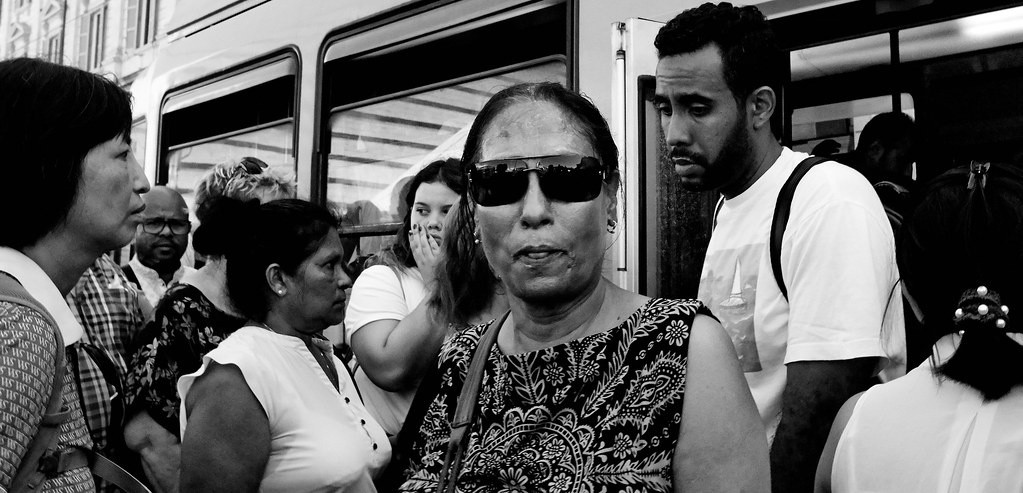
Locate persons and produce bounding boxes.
[651,3,909,493]
[0,0,1022,493]
[388,81,772,493]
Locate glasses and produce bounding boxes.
[467,154,610,203]
[143,216,191,236]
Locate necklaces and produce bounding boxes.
[260,320,340,392]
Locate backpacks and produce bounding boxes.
[770,156,938,375]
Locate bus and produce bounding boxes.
[127,1,1023,301]
